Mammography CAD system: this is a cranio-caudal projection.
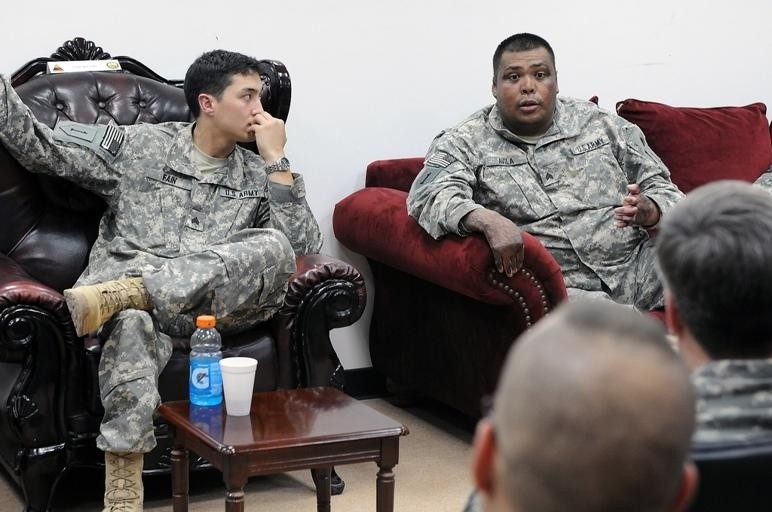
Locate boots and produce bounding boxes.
[63,276,155,338]
[101,451,144,512]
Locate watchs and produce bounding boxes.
[266,157,290,176]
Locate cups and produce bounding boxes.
[220,355,258,417]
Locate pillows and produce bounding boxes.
[616,97,772,194]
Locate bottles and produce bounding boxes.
[188,314,223,407]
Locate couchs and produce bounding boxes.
[332,98,772,418]
